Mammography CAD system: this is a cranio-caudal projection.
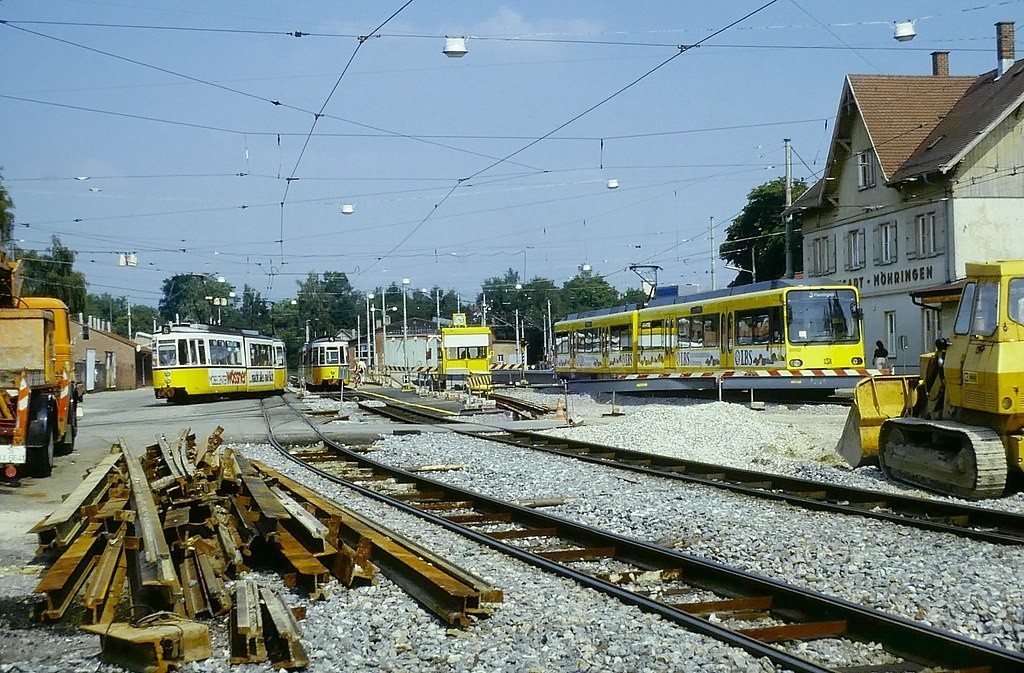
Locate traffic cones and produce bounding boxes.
[555,398,565,420]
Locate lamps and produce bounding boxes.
[340,204,354,215]
[441,38,468,59]
[607,178,619,190]
[893,23,917,42]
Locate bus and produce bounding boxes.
[136,314,287,403]
[555,278,867,401]
[298,335,350,391]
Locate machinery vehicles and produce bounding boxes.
[0,250,78,484]
[834,260,1024,500]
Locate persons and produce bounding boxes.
[352,358,366,388]
[460,348,471,359]
[872,340,889,365]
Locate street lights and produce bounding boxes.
[398,277,410,375]
[370,307,397,365]
[367,294,374,370]
[724,247,756,285]
